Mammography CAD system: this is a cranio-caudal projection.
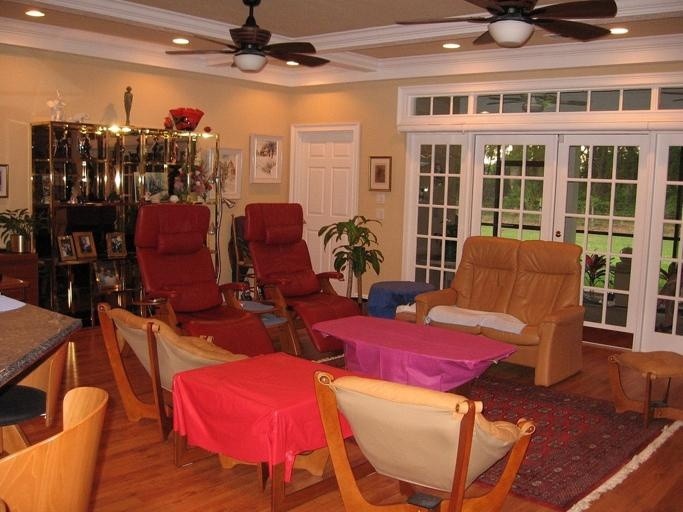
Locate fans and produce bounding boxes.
[390,1,618,50]
[164,1,329,74]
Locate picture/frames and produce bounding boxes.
[93,259,121,290]
[71,230,96,258]
[105,231,126,258]
[133,171,169,202]
[248,132,285,184]
[56,235,75,260]
[0,164,9,199]
[368,154,392,192]
[206,147,242,201]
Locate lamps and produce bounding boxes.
[486,1,535,49]
[229,0,272,74]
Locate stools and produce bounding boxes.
[606,350,683,427]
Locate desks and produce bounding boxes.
[362,281,436,319]
[172,351,376,511]
[0,293,81,388]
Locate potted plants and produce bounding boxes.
[317,214,385,315]
[0,209,46,254]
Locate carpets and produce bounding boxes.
[435,374,683,512]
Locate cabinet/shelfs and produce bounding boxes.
[0,254,37,306]
[32,123,220,328]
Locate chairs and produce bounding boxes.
[245,202,363,363]
[230,215,257,301]
[0,336,65,455]
[311,365,534,511]
[133,202,277,358]
[0,386,108,512]
[95,302,247,440]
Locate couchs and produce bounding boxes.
[414,237,586,387]
